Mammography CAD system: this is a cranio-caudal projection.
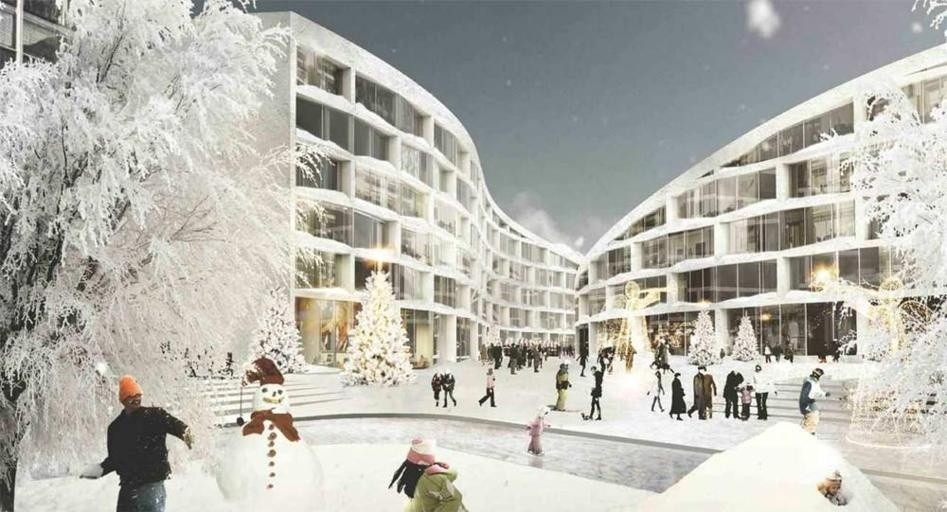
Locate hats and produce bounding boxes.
[407,437,435,466]
[119,375,143,401]
[240,358,283,385]
